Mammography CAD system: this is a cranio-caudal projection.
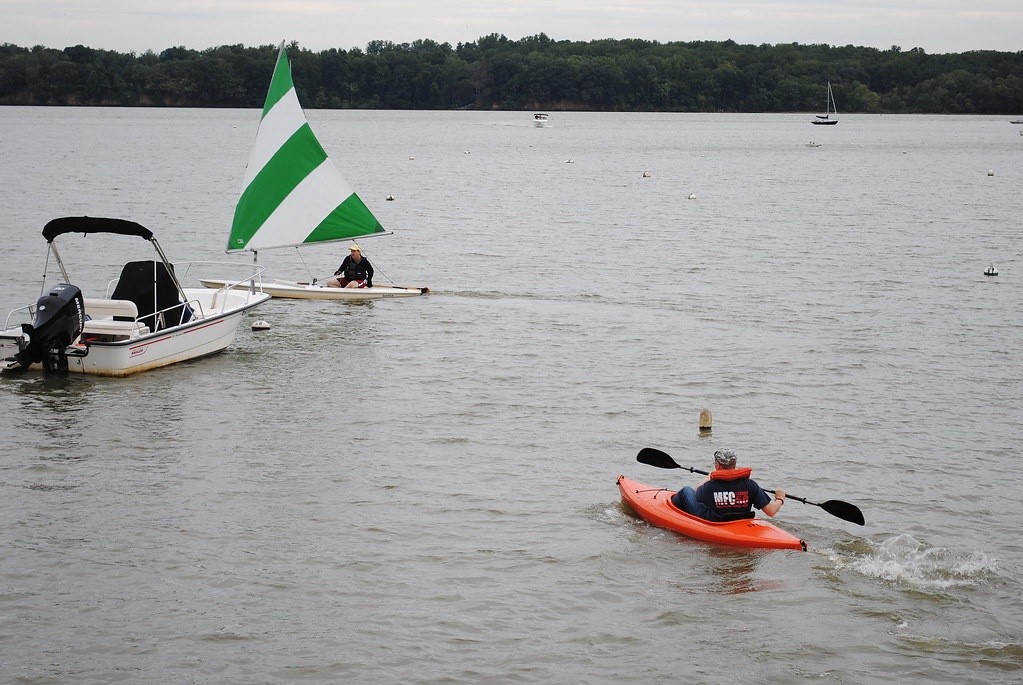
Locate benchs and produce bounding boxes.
[84,298,150,339]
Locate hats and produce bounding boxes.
[713,448,737,468]
[349,244,362,251]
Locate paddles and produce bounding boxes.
[635,448,866,527]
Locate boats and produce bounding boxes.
[532,112,549,129]
[0,214,279,381]
[610,472,808,559]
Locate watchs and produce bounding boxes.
[775,496,784,505]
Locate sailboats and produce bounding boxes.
[199,39,430,301]
[812,81,839,126]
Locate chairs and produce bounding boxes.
[718,511,755,522]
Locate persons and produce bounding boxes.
[809,141,819,150]
[535,115,545,120]
[671,447,786,522]
[327,245,374,289]
